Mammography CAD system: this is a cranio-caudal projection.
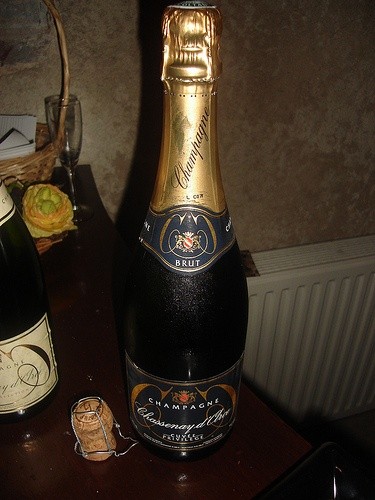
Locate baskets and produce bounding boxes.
[1,123,55,193]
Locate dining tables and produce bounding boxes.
[0,167,366,500]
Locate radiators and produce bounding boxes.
[227,234,375,426]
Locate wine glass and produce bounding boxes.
[46,95,94,223]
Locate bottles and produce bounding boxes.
[122,5,248,460]
[0,180,61,423]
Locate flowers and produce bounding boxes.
[21,181,76,238]
